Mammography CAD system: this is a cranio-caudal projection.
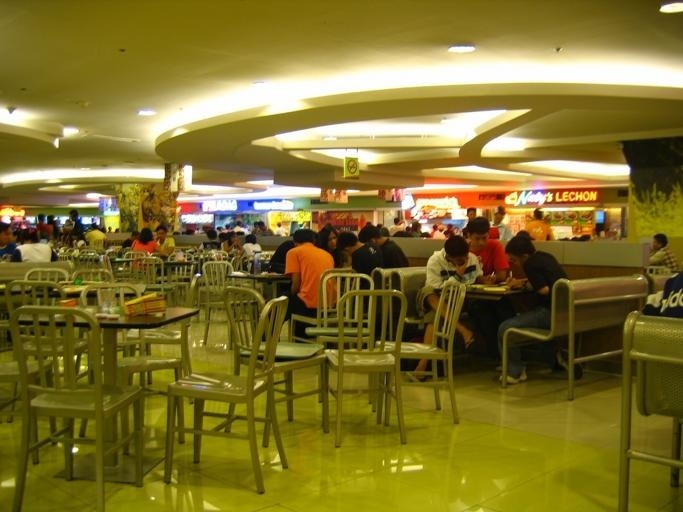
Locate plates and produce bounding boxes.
[467,284,497,290]
[483,287,507,294]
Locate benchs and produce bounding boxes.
[371,267,428,339]
[616,310,682,511]
[501,273,649,401]
[389,267,469,341]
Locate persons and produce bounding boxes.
[131,229,161,270]
[155,226,175,251]
[256,222,273,235]
[285,229,338,319]
[497,236,569,383]
[11,228,59,264]
[431,226,438,236]
[85,223,106,247]
[251,222,258,234]
[462,208,476,235]
[410,235,483,379]
[222,224,232,233]
[36,214,50,238]
[411,221,422,238]
[389,217,405,236]
[466,217,509,283]
[275,223,287,236]
[68,210,84,246]
[336,231,382,274]
[63,219,73,237]
[318,226,343,268]
[234,221,245,232]
[0,221,16,261]
[118,231,140,259]
[490,206,511,241]
[269,230,321,278]
[243,235,261,255]
[201,229,218,249]
[47,215,59,237]
[650,233,678,271]
[444,224,455,238]
[358,224,409,267]
[217,233,245,260]
[525,209,551,241]
[433,224,446,238]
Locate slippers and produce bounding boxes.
[463,331,474,351]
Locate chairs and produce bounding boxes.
[79,282,186,445]
[121,251,146,281]
[223,286,327,448]
[319,274,375,405]
[4,280,95,445]
[168,252,195,291]
[324,290,408,448]
[9,305,145,512]
[373,277,466,425]
[202,261,258,349]
[134,257,165,301]
[126,273,202,386]
[12,268,70,362]
[72,268,115,375]
[164,295,290,494]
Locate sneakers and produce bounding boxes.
[499,368,528,384]
